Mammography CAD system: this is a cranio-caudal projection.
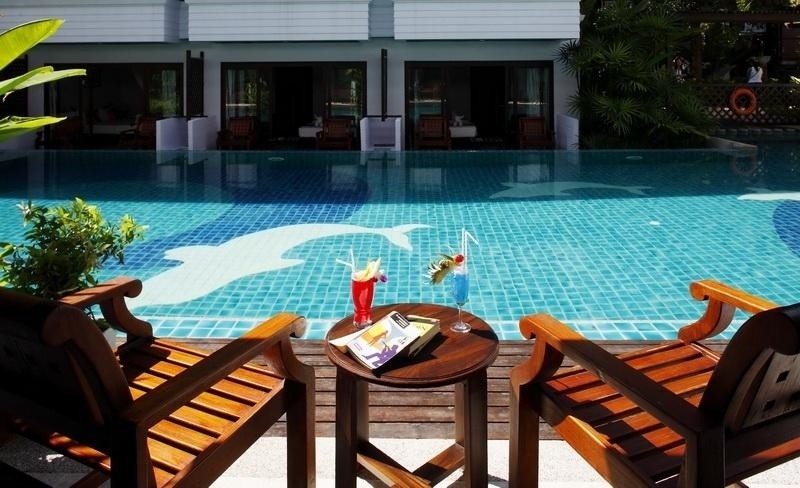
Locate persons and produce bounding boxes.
[673,56,688,82]
[746,59,764,84]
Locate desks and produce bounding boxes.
[298,126,324,150]
[449,125,477,150]
[325,302,499,488]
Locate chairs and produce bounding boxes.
[120,112,162,148]
[413,114,452,150]
[216,117,259,150]
[316,115,355,151]
[508,280,800,488]
[52,116,85,150]
[517,115,554,149]
[0,275,315,487]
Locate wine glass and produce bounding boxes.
[449,262,471,333]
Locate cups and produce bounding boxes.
[351,269,375,328]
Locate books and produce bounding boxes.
[328,310,441,371]
